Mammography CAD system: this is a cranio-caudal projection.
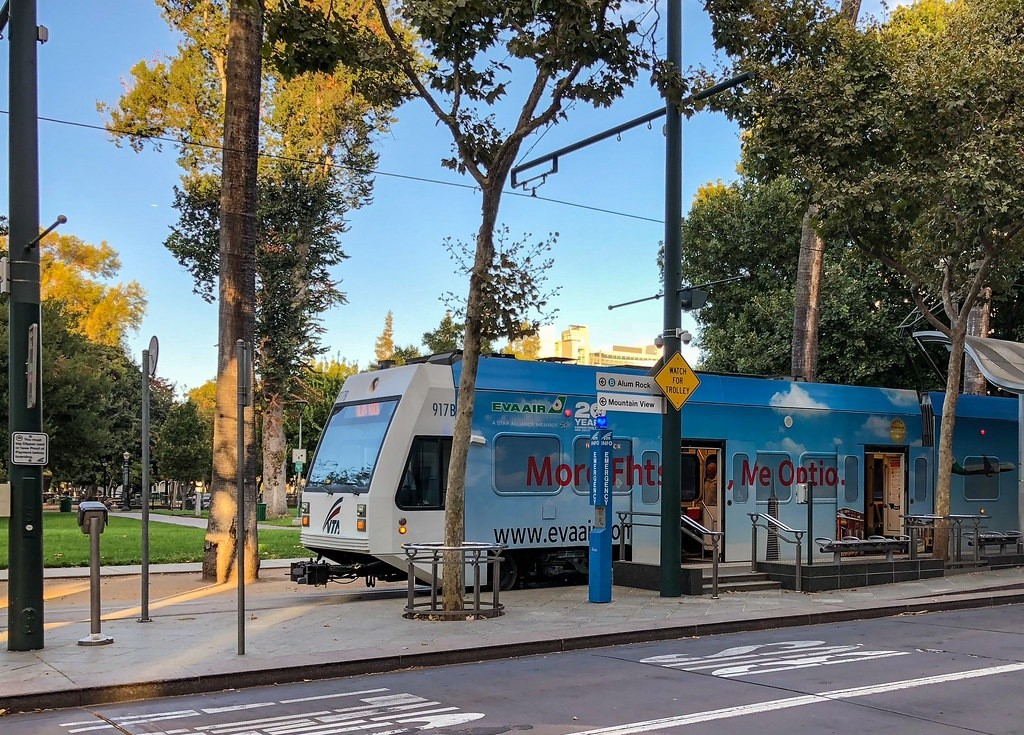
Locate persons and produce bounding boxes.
[694,463,718,506]
[401,457,417,490]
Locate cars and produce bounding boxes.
[44,488,210,513]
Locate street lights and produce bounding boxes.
[294,399,309,517]
[121,451,132,510]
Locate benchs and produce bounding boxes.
[814,534,912,563]
[962,530,1023,554]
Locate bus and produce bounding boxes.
[286,347,1024,588]
